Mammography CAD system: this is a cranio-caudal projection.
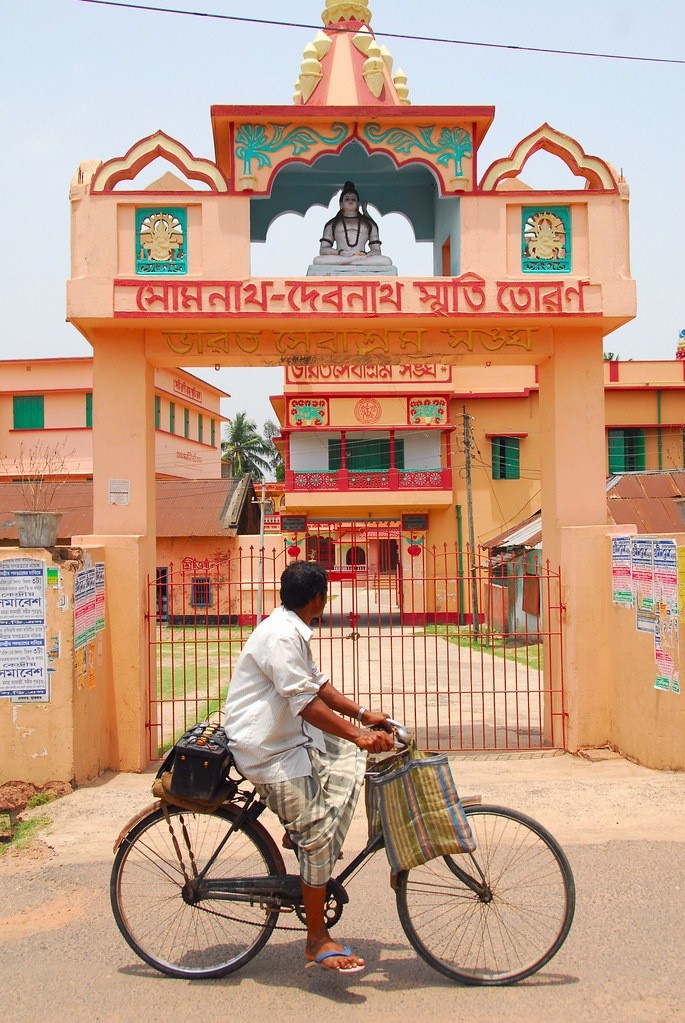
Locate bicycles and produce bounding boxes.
[109,718,576,981]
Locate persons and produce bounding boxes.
[313,181,392,266]
[225,560,395,973]
[536,220,554,258]
[151,223,169,260]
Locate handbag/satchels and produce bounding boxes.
[369,750,477,874]
[361,738,418,853]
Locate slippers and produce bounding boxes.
[282,832,345,859]
[304,945,365,973]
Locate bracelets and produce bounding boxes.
[357,707,366,721]
